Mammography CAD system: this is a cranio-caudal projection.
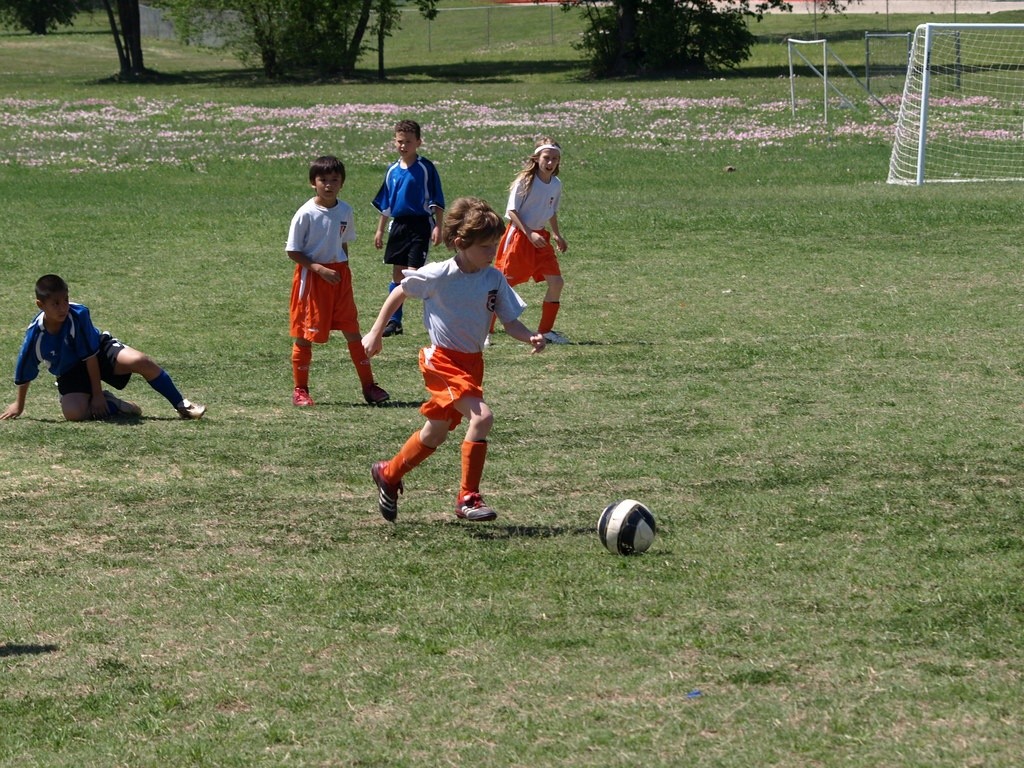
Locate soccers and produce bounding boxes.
[598,498,658,555]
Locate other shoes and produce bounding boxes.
[175,399,206,420]
[105,389,142,416]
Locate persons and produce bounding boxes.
[361,197,545,524]
[371,120,448,337]
[0,274,207,422]
[481,136,569,347]
[284,156,390,408]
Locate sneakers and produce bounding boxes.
[371,462,403,522]
[455,492,497,521]
[483,332,493,347]
[543,331,568,344]
[292,387,313,406]
[382,319,403,337]
[362,383,389,404]
